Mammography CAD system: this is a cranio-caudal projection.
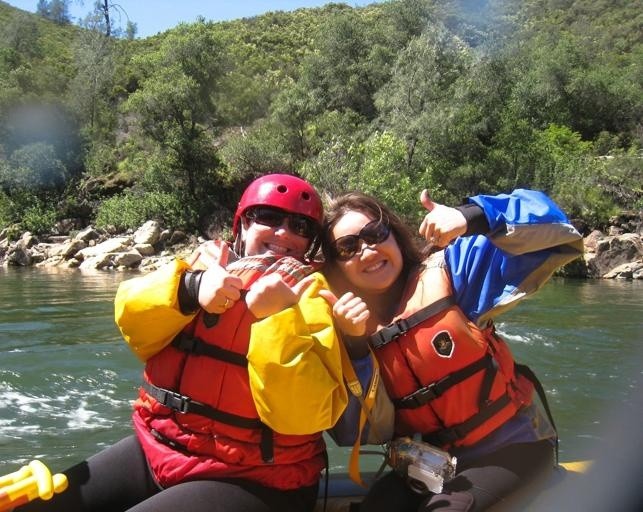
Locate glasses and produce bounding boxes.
[246,207,319,239]
[326,218,391,262]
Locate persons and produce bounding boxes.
[316,186,585,511]
[8,173,348,512]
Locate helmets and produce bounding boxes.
[232,173,324,243]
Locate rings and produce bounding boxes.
[223,299,228,308]
